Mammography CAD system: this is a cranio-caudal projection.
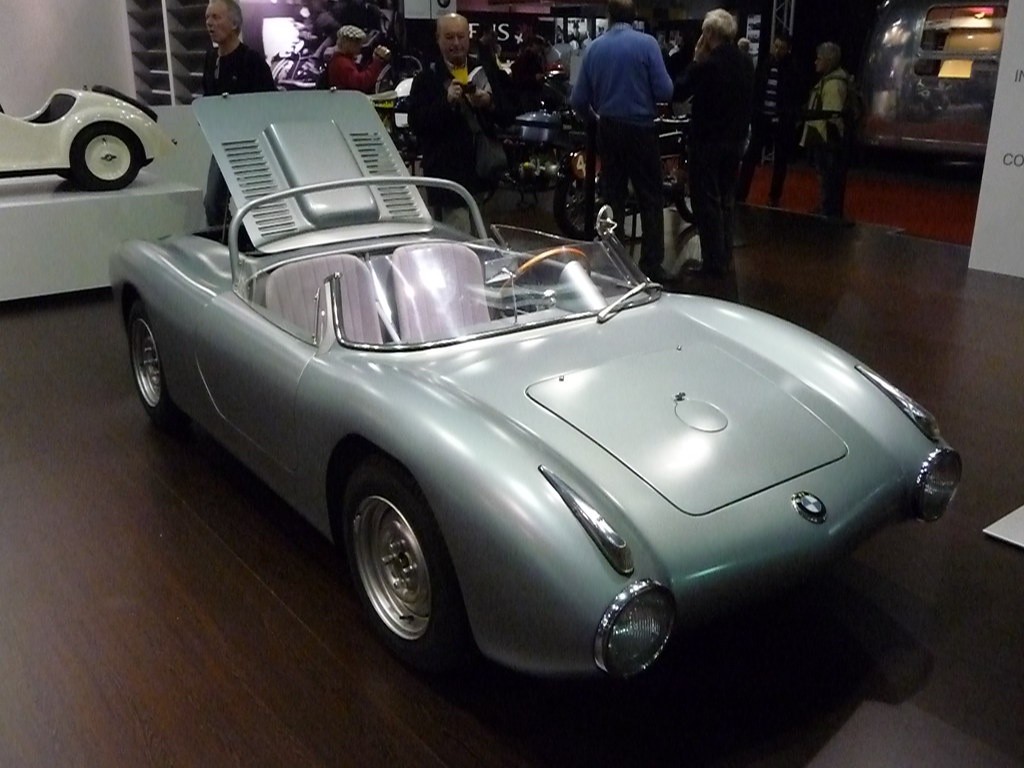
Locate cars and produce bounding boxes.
[108,85,962,686]
[0,84,179,190]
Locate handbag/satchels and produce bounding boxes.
[473,133,506,196]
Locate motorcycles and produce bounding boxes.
[553,94,705,242]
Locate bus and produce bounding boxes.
[860,0,1011,155]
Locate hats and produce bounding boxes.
[336,25,366,39]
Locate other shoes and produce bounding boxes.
[643,265,676,284]
[679,259,717,281]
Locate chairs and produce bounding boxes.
[264,254,384,345]
[391,242,490,342]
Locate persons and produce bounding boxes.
[737,37,751,56]
[570,0,676,283]
[799,42,855,219]
[202,0,274,226]
[738,33,810,208]
[470,23,551,179]
[326,25,391,90]
[407,13,515,237]
[674,8,755,278]
